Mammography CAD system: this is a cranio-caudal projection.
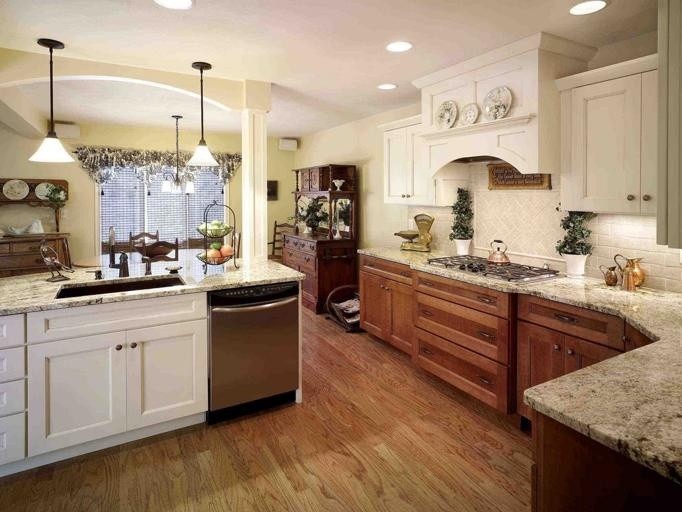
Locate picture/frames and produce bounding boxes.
[486,162,552,192]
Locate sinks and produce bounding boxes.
[54,273,187,300]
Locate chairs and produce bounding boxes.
[141,237,179,263]
[62,237,72,269]
[229,231,242,259]
[129,229,160,252]
[268,220,299,262]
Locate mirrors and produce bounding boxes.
[315,198,352,233]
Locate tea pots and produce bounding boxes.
[599,264,617,286]
[618,268,640,292]
[487,239,511,265]
[614,254,645,287]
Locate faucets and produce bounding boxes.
[108,225,130,277]
[141,256,152,276]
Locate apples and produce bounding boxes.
[207,249,221,262]
[220,245,234,257]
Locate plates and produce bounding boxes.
[482,86,512,120]
[461,103,480,124]
[2,179,31,201]
[35,182,56,201]
[433,101,457,129]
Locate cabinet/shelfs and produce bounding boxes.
[377,114,468,207]
[553,53,657,216]
[26,290,210,474]
[358,252,415,357]
[517,291,626,433]
[1,309,27,478]
[282,164,357,315]
[0,231,73,279]
[527,409,681,512]
[414,268,516,425]
[627,317,655,354]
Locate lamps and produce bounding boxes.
[161,114,196,195]
[278,137,298,152]
[27,38,76,164]
[184,61,221,168]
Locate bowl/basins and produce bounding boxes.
[133,242,175,259]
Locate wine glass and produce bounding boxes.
[333,180,345,191]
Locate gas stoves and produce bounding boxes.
[426,253,560,284]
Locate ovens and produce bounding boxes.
[206,282,300,426]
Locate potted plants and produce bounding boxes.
[48,183,69,208]
[295,199,329,236]
[555,202,598,276]
[448,187,475,257]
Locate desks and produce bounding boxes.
[74,248,207,269]
